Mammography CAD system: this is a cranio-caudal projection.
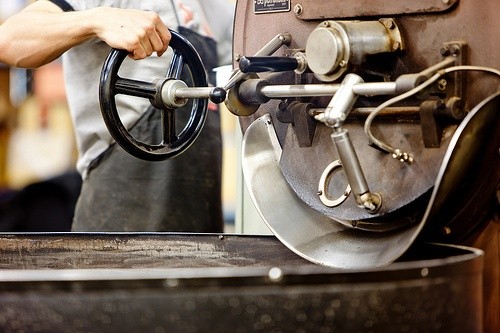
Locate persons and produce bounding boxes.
[1,0,238,234]
